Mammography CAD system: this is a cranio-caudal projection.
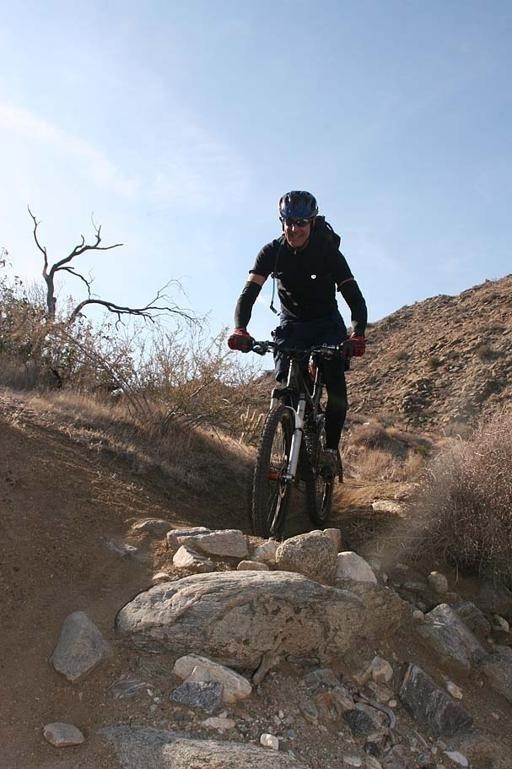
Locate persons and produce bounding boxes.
[232,190,367,482]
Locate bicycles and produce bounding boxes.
[232,334,348,539]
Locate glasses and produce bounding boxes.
[285,218,313,227]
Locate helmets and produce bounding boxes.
[278,190,319,220]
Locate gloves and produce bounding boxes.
[349,331,366,357]
[228,327,253,352]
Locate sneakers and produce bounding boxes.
[319,448,340,481]
